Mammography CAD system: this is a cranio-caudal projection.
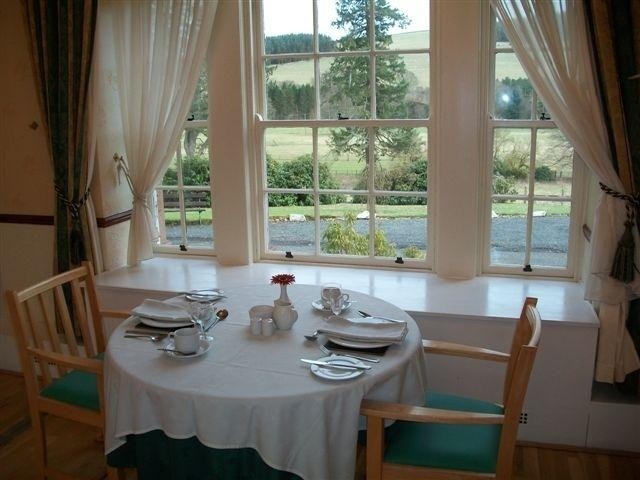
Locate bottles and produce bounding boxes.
[262,317,273,338]
[250,316,262,336]
[273,303,298,331]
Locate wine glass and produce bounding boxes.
[320,284,343,310]
[330,293,342,315]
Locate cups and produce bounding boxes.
[167,328,203,354]
[320,287,349,302]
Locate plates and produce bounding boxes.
[164,339,211,359]
[312,299,352,312]
[186,289,223,303]
[328,333,393,349]
[310,356,366,381]
[139,317,195,329]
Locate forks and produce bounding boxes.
[318,344,380,363]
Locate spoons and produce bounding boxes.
[204,308,228,335]
[304,330,318,341]
[357,310,404,323]
[124,334,168,342]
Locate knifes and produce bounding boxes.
[299,357,372,371]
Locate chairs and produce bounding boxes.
[5,260,131,479]
[358,297,543,479]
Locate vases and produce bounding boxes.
[274,284,292,303]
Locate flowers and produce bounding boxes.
[269,273,296,286]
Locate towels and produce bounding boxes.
[131,297,194,322]
[317,315,409,346]
